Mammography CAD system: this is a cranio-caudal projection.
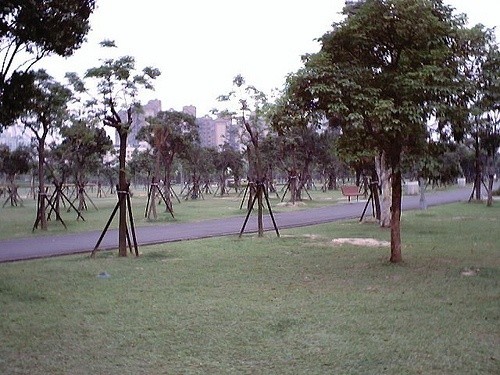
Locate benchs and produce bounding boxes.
[340,185,363,201]
